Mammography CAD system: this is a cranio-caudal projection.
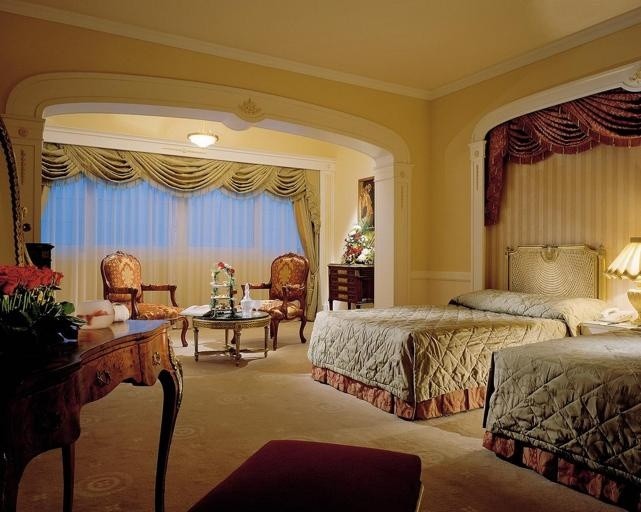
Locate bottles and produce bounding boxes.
[239,282,255,317]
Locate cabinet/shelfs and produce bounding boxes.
[325,262,374,312]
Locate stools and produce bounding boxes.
[188,437,425,511]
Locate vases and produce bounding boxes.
[18,333,46,373]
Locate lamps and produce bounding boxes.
[602,236,641,327]
[186,131,219,149]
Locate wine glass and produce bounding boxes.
[253,299,262,316]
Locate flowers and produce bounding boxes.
[0,265,83,339]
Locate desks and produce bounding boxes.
[189,305,272,365]
[0,320,183,511]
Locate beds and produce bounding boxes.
[307,242,606,420]
[480,318,640,511]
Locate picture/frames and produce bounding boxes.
[357,174,375,232]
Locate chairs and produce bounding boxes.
[99,251,188,349]
[242,251,309,350]
